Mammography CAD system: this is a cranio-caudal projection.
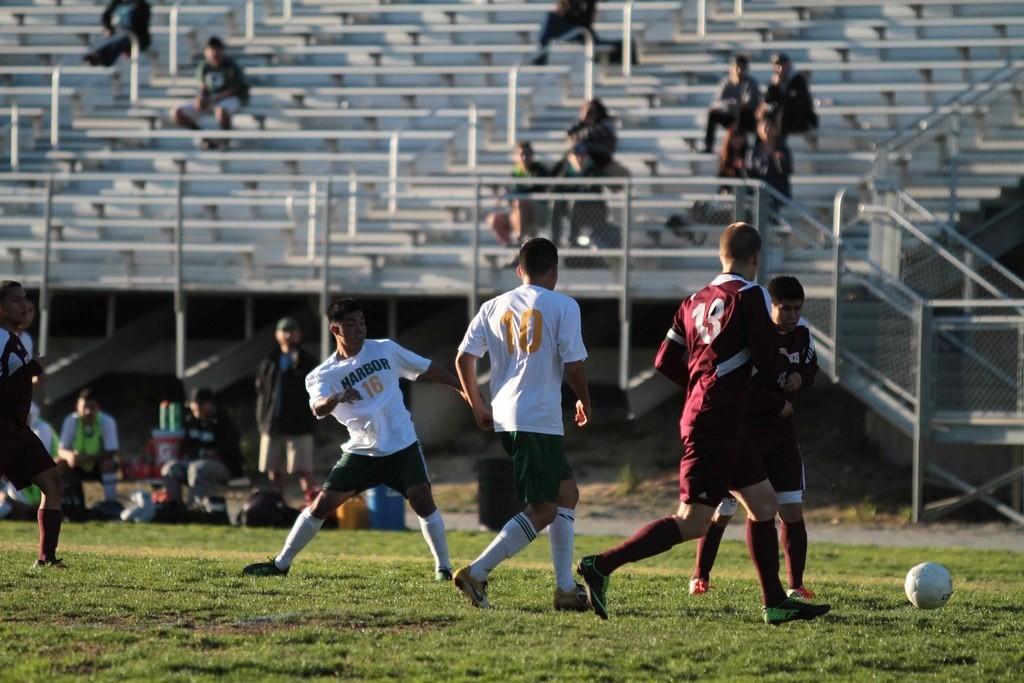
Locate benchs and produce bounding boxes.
[0,1,1024,331]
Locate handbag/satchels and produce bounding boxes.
[238,480,407,531]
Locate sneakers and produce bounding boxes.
[453,565,491,607]
[764,598,831,624]
[576,554,612,620]
[553,584,592,612]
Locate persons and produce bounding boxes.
[690,275,817,602]
[528,0,602,67]
[241,298,486,584]
[169,36,252,150]
[489,53,817,248]
[81,0,154,66]
[449,237,594,612]
[0,279,319,569]
[575,222,830,628]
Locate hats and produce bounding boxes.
[277,318,300,330]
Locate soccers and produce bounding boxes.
[904,562,953,610]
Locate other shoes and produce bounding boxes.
[31,559,69,569]
[242,553,290,577]
[437,567,454,583]
[689,578,716,595]
[786,586,817,599]
